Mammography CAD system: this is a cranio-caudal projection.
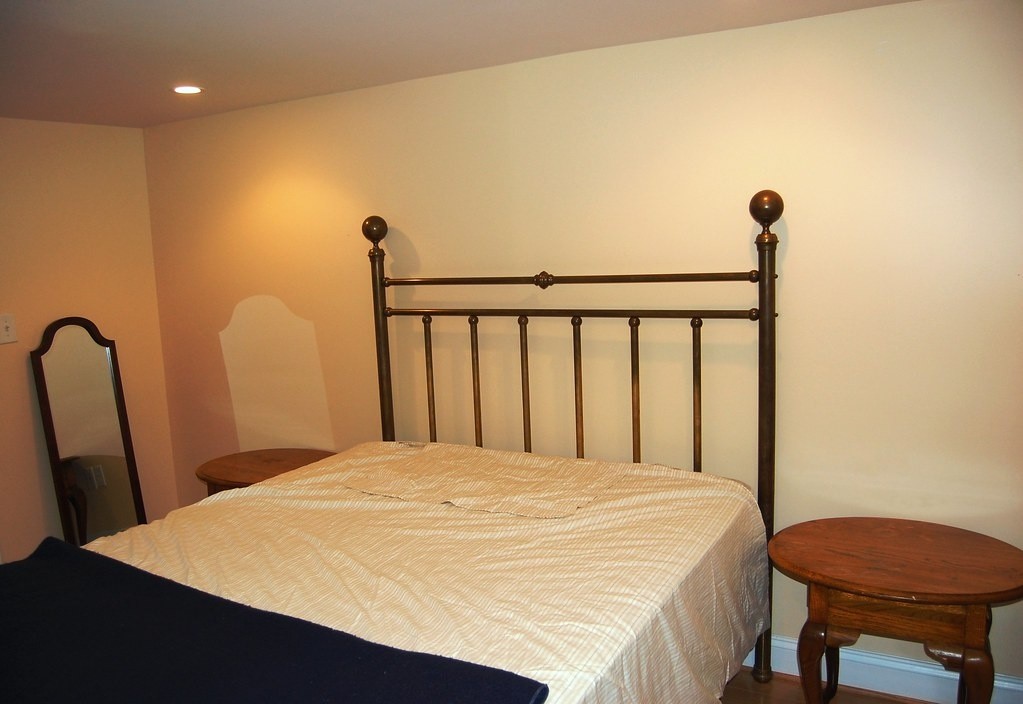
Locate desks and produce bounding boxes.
[768,518,1023,704]
[59,456,89,547]
[195,448,340,498]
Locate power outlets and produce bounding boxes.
[0,313,19,346]
[92,465,107,489]
[84,467,98,490]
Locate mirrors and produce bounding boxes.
[29,316,148,544]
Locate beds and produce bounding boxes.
[0,189,784,704]
